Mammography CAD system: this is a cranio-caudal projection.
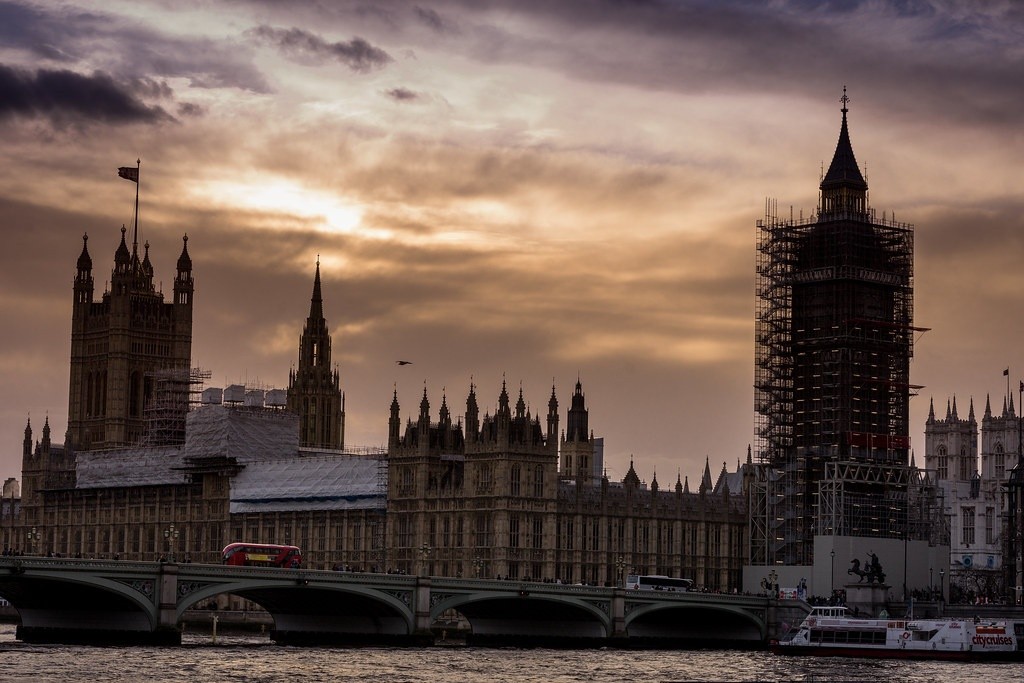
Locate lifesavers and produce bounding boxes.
[903,631,909,639]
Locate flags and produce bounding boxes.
[1003,369,1008,375]
[119,167,139,182]
[1020,382,1024,391]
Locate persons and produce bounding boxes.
[871,553,880,573]
[634,584,676,597]
[156,554,166,562]
[2,548,120,567]
[686,584,769,602]
[510,575,613,592]
[332,564,366,572]
[497,574,509,586]
[388,568,406,575]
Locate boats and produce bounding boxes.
[770,606,1023,664]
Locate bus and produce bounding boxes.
[626,575,694,592]
[220,542,301,569]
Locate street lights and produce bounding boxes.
[929,567,934,600]
[939,568,945,597]
